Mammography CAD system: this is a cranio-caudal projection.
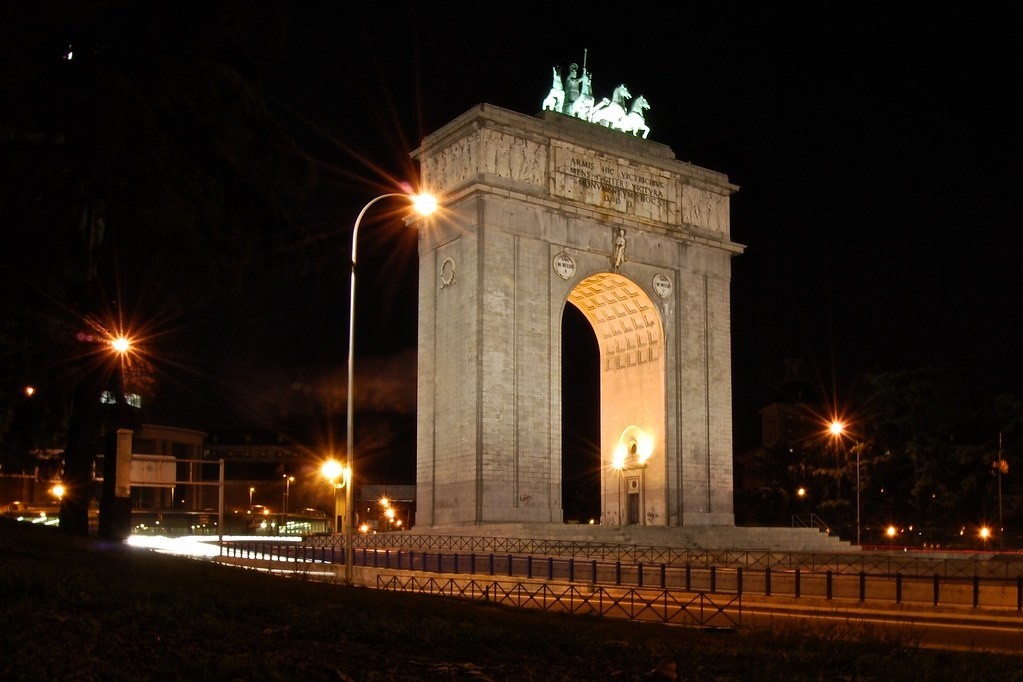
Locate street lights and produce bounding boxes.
[828,418,860,546]
[344,190,440,587]
[249,486,254,507]
[283,472,295,513]
[322,458,343,534]
